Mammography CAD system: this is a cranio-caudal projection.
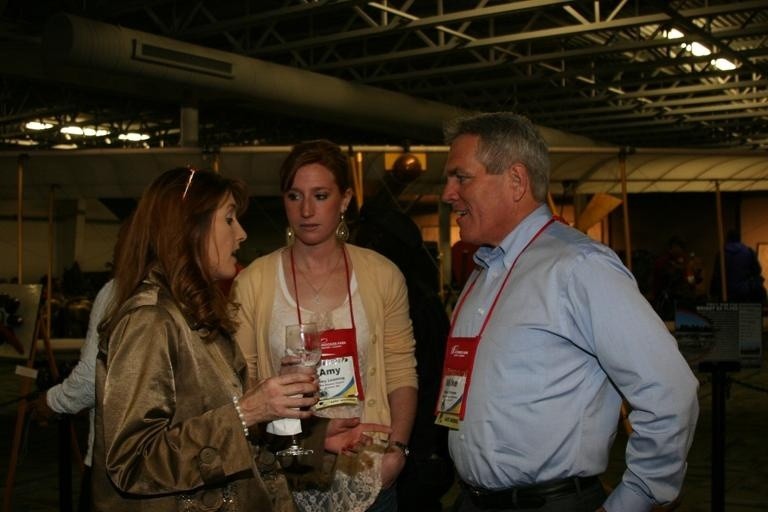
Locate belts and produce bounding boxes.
[456,475,601,507]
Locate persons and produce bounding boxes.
[90,166,393,512]
[225,139,418,512]
[447,111,701,512]
[708,230,768,304]
[27,209,120,512]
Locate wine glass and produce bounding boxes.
[274,323,323,457]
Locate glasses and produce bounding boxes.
[182,168,202,199]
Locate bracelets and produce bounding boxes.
[385,439,410,459]
[230,397,250,438]
